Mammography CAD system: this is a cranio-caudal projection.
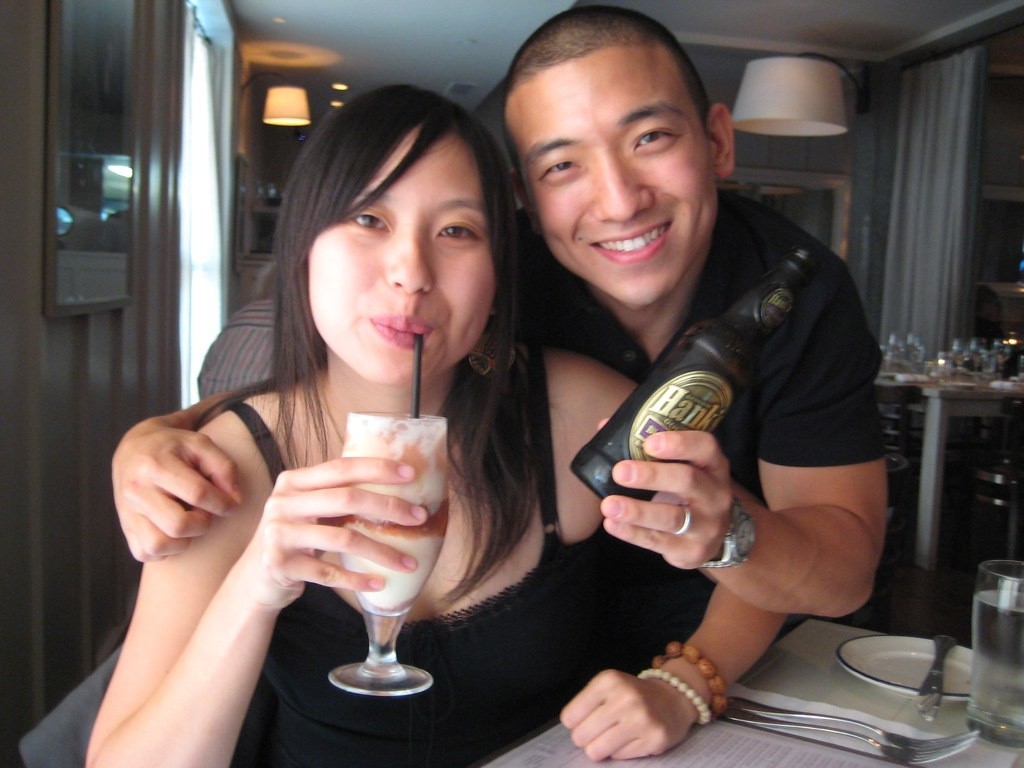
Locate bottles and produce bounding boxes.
[570,247,819,505]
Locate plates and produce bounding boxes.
[838,634,974,702]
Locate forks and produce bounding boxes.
[726,696,978,748]
[722,710,973,764]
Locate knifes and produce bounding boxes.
[916,635,957,721]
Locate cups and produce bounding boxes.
[967,561,1024,748]
[882,332,1016,388]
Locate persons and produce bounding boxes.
[112,5,890,633]
[84,86,789,768]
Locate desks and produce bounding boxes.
[481,620,1024,768]
[875,358,1024,569]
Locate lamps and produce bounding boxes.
[241,71,312,126]
[730,52,861,137]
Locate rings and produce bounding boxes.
[674,503,692,536]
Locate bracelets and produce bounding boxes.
[635,641,728,724]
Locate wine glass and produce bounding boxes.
[328,411,449,696]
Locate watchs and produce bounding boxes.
[698,493,755,569]
